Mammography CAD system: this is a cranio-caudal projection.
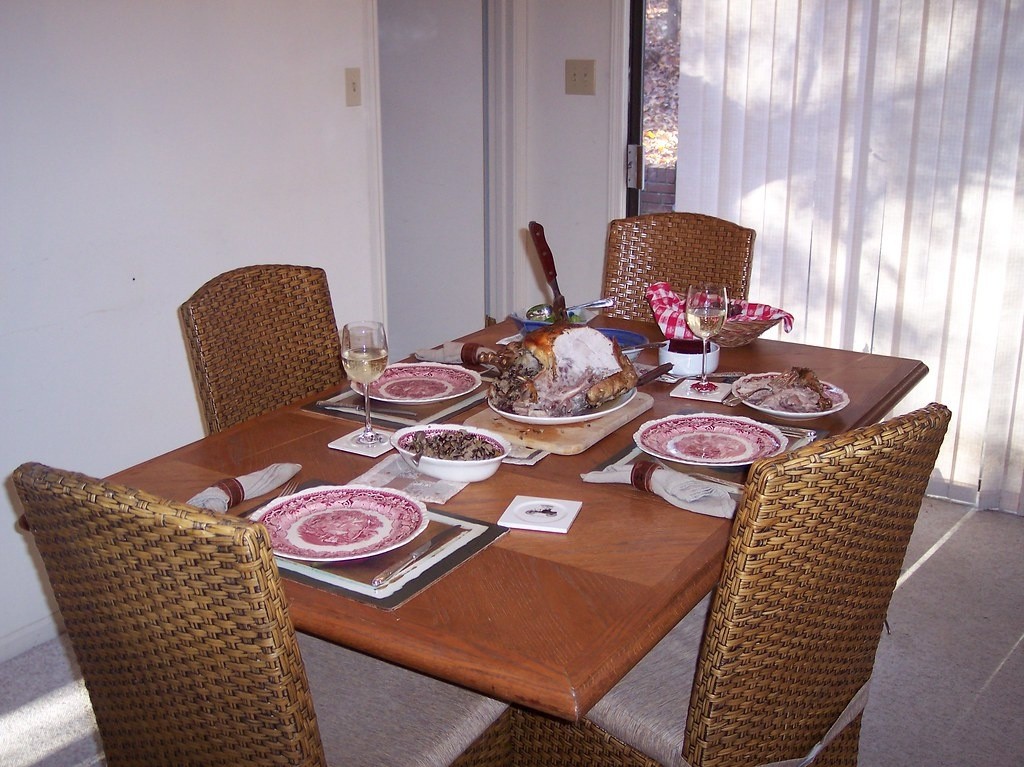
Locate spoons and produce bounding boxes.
[409,431,428,467]
[526,298,613,320]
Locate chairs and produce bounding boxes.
[600,212,758,322]
[13,461,513,767]
[515,401,952,767]
[178,264,348,437]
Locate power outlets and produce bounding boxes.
[564,60,596,94]
[345,67,362,107]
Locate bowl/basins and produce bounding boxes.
[510,313,598,335]
[658,338,720,376]
[390,423,511,482]
[595,328,649,363]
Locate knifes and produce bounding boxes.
[372,524,461,587]
[316,401,417,416]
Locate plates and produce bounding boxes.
[732,371,851,421]
[250,485,430,561]
[496,495,582,534]
[327,426,396,458]
[670,380,731,403]
[487,386,637,426]
[633,412,789,467]
[350,362,482,406]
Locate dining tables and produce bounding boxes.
[101,311,930,726]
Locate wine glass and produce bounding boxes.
[341,321,389,447]
[685,284,727,394]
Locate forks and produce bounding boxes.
[244,481,299,521]
[656,371,745,384]
[723,370,798,407]
[654,458,744,488]
[479,366,495,376]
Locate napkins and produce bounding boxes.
[185,462,302,516]
[414,340,501,374]
[580,460,736,519]
